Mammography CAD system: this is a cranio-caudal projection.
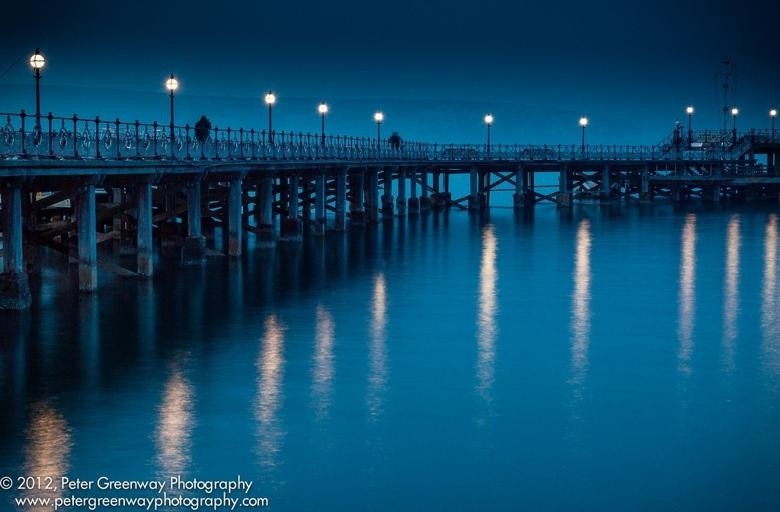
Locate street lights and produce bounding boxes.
[166,73,178,144]
[770,107,776,144]
[265,90,276,145]
[374,110,383,150]
[687,105,694,150]
[485,114,493,156]
[30,46,46,146]
[580,115,588,154]
[730,108,739,146]
[318,100,329,147]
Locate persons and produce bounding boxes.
[194,115,211,154]
[388,132,404,155]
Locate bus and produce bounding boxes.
[436,148,480,160]
[520,149,555,160]
